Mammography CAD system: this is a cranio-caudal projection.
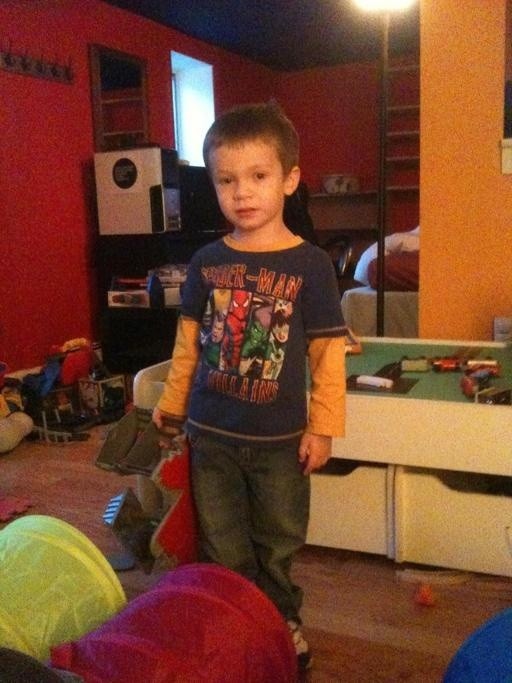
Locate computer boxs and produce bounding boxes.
[94,147,182,236]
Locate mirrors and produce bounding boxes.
[90,42,151,151]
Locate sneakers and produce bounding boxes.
[286,619,310,667]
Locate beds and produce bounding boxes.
[342,285,419,340]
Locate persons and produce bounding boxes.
[150,98,349,672]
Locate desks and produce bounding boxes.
[132,334,512,578]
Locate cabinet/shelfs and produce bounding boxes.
[380,65,421,192]
[307,190,383,293]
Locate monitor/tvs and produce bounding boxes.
[179,165,226,232]
